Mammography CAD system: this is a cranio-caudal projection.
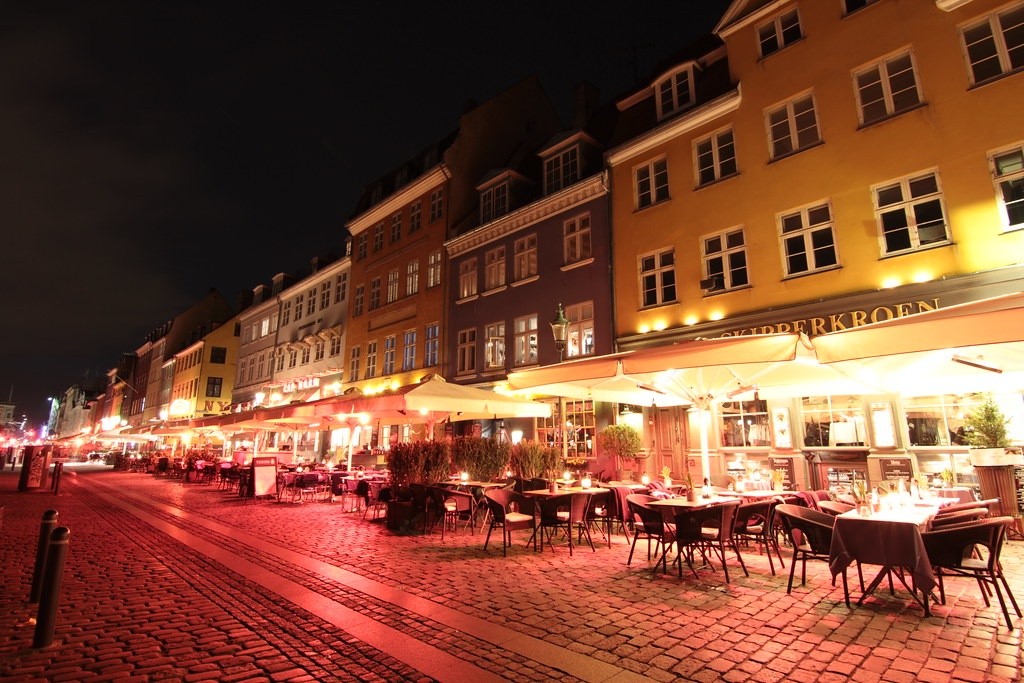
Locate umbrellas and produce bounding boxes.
[314,373,552,442]
[506,331,858,486]
[137,386,376,472]
[808,290,1022,393]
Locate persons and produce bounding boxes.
[240,446,247,451]
[310,458,318,471]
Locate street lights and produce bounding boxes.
[548,303,571,477]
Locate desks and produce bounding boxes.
[645,494,742,576]
[435,480,507,536]
[828,492,960,619]
[522,486,610,557]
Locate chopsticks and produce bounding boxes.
[849,481,866,504]
[661,467,670,478]
[548,472,556,483]
[771,469,784,485]
[681,472,694,491]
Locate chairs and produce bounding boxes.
[80,452,1024,631]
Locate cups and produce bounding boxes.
[664,480,671,485]
[855,502,870,514]
[550,484,558,493]
[687,492,696,501]
[860,507,869,517]
[775,484,782,493]
